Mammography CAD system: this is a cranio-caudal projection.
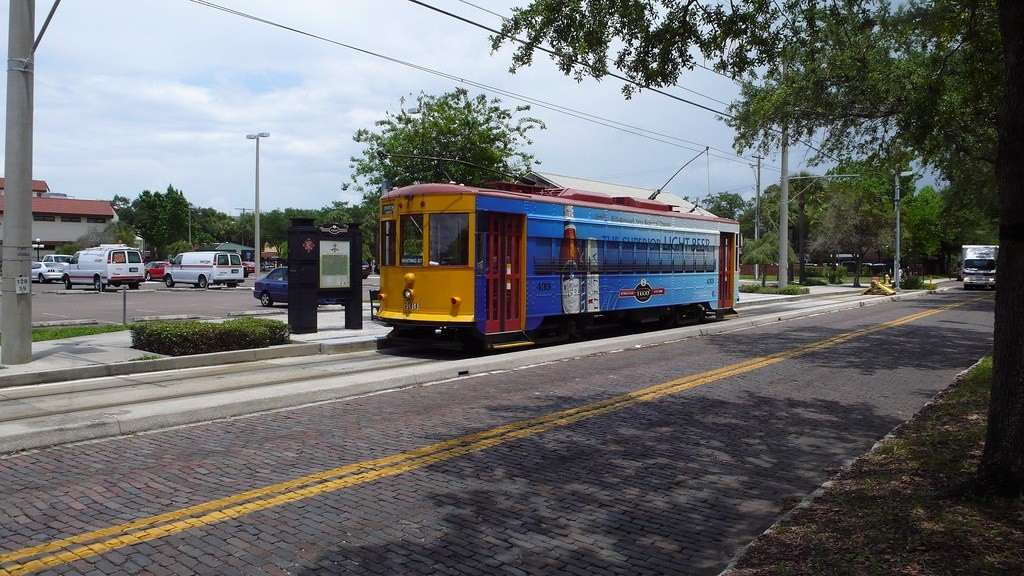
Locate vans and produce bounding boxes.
[61,247,146,292]
[163,251,245,288]
[43,254,73,263]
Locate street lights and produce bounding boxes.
[247,132,272,281]
[32,238,44,262]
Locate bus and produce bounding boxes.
[371,178,744,354]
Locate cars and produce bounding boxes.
[362,260,370,279]
[242,261,255,277]
[145,261,170,281]
[253,266,345,307]
[31,262,65,284]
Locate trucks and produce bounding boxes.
[962,244,999,291]
